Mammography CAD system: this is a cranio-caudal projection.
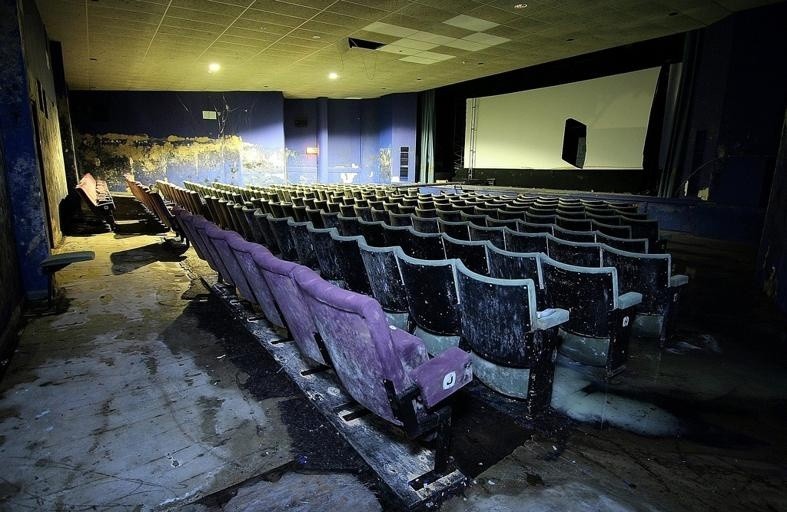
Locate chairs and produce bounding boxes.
[69,172,118,233]
[125,172,785,504]
